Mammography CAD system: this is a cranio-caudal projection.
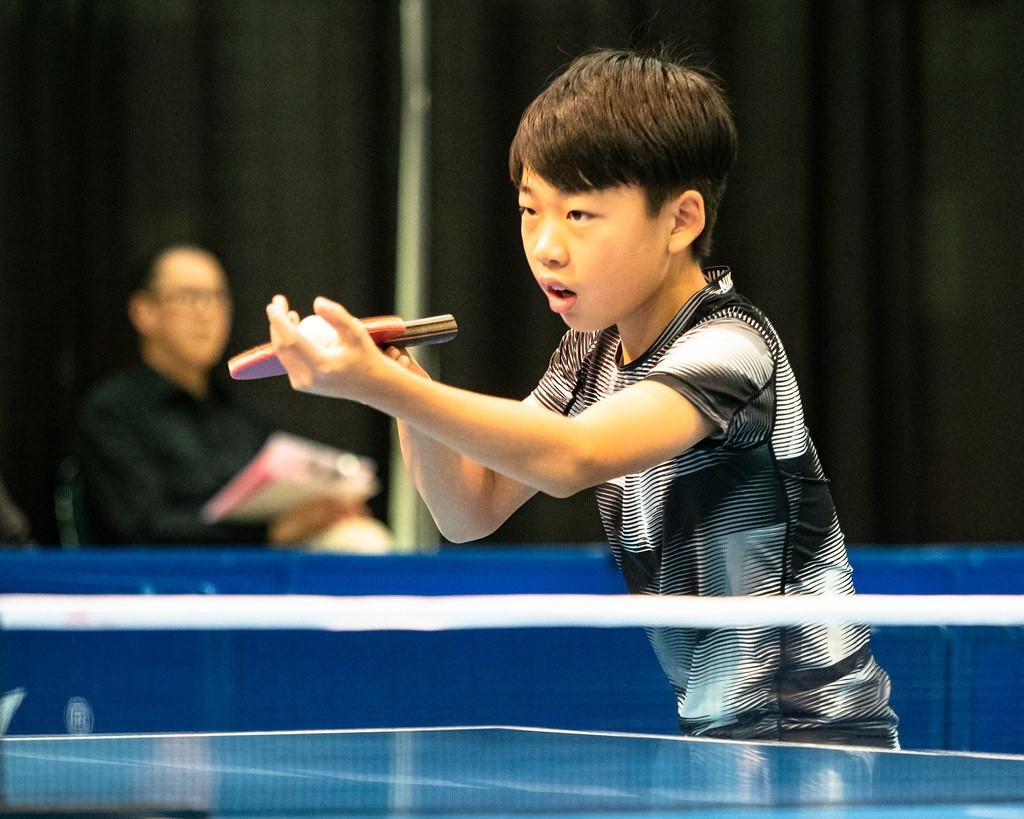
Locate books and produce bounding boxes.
[202,438,382,524]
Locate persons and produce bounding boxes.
[263,51,906,762]
[70,235,404,557]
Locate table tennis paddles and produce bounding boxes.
[227,312,456,382]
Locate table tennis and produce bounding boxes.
[300,313,339,352]
